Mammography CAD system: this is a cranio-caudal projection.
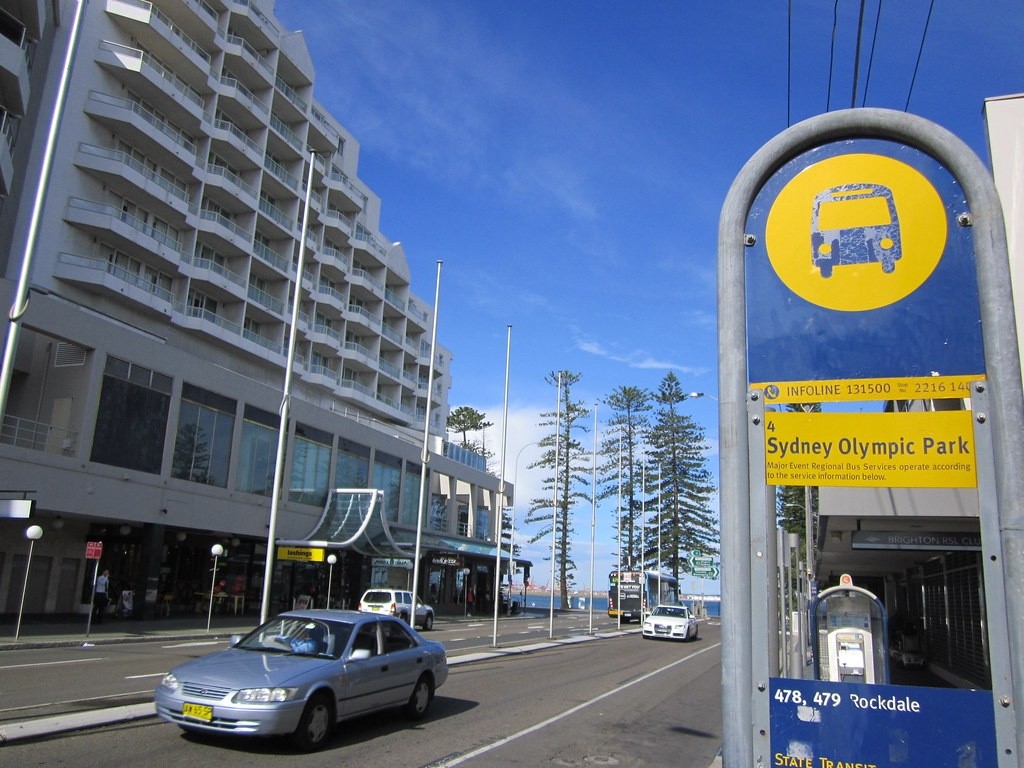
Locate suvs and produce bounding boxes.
[359,589,435,631]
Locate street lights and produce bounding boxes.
[505,436,565,616]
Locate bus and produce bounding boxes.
[608,570,680,623]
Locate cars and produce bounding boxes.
[643,605,699,641]
[154,608,447,749]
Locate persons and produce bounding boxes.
[466,587,475,618]
[499,595,504,613]
[267,622,319,655]
[485,591,491,614]
[214,578,227,594]
[90,568,109,625]
